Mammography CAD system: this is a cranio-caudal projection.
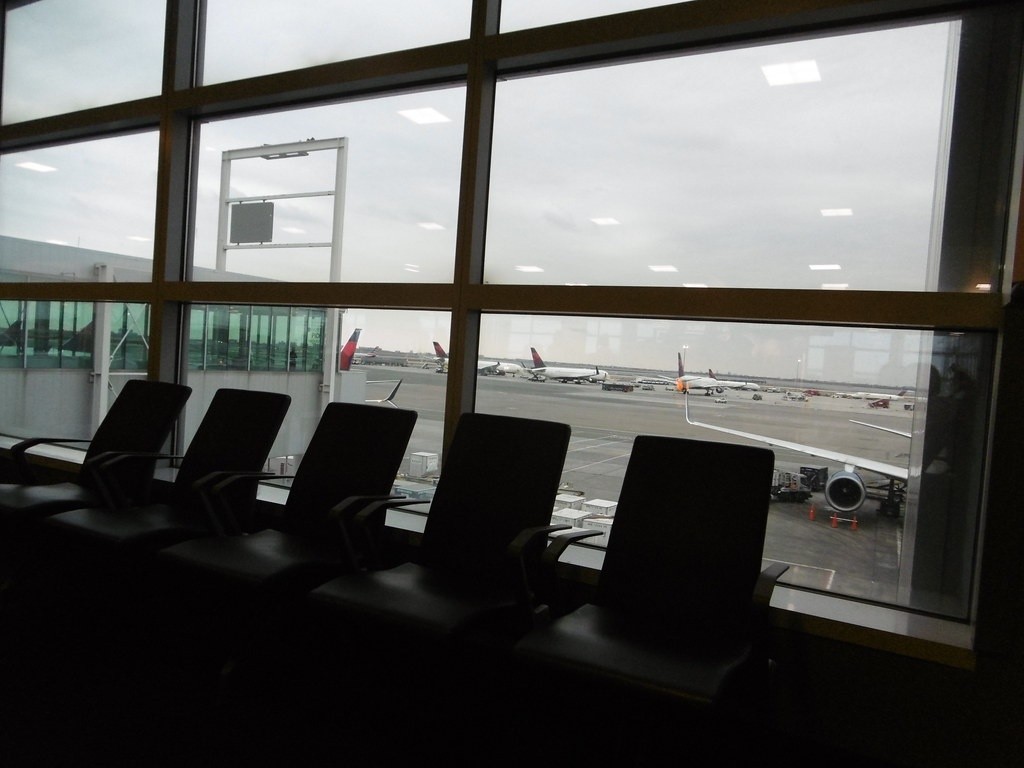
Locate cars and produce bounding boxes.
[715,397,727,403]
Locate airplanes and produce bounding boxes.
[836,388,915,401]
[432,342,611,384]
[685,388,912,518]
[340,328,408,408]
[657,353,761,396]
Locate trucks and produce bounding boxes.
[770,468,813,504]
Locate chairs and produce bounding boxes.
[307,411,574,637]
[0,379,193,523]
[43,386,293,551]
[509,434,791,711]
[150,402,418,589]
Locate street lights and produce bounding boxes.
[795,360,800,387]
[682,345,688,370]
